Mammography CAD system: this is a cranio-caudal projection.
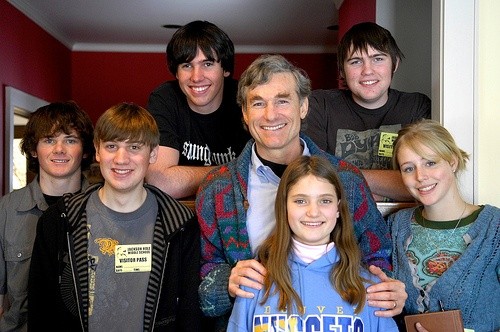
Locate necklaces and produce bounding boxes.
[424,202,466,253]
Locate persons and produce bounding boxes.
[145,20,252,199]
[226,156,399,332]
[195,55,408,316]
[26,103,202,332]
[299,22,432,202]
[0,101,94,332]
[387,118,500,332]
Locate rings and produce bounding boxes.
[393,301,397,308]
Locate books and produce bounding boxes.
[404,310,464,332]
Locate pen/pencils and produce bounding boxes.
[438,300,444,312]
